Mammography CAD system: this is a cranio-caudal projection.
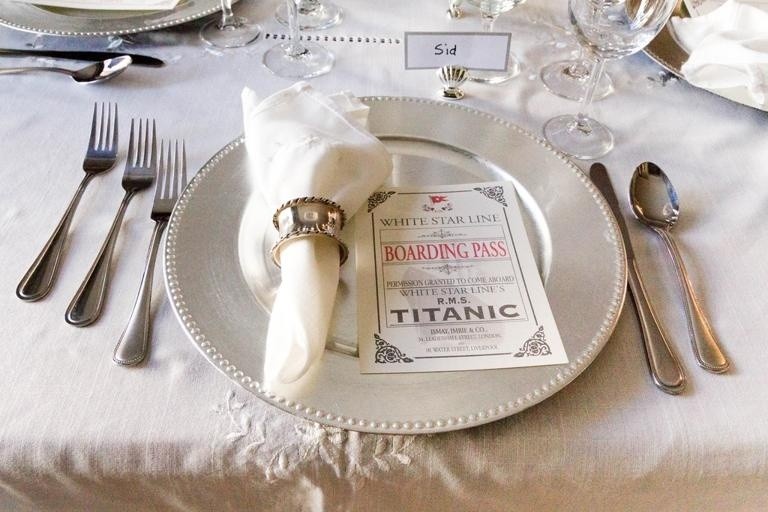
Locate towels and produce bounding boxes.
[669,0,768,100]
[237,82,393,392]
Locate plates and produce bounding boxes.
[0,0,242,36]
[161,95,629,435]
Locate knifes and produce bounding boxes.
[0,47,163,69]
[588,163,688,393]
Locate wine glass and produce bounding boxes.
[466,0,677,160]
[200,0,343,80]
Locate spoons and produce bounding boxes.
[629,162,731,373]
[0,55,133,85]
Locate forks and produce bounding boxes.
[113,138,187,367]
[15,101,117,304]
[66,118,158,329]
